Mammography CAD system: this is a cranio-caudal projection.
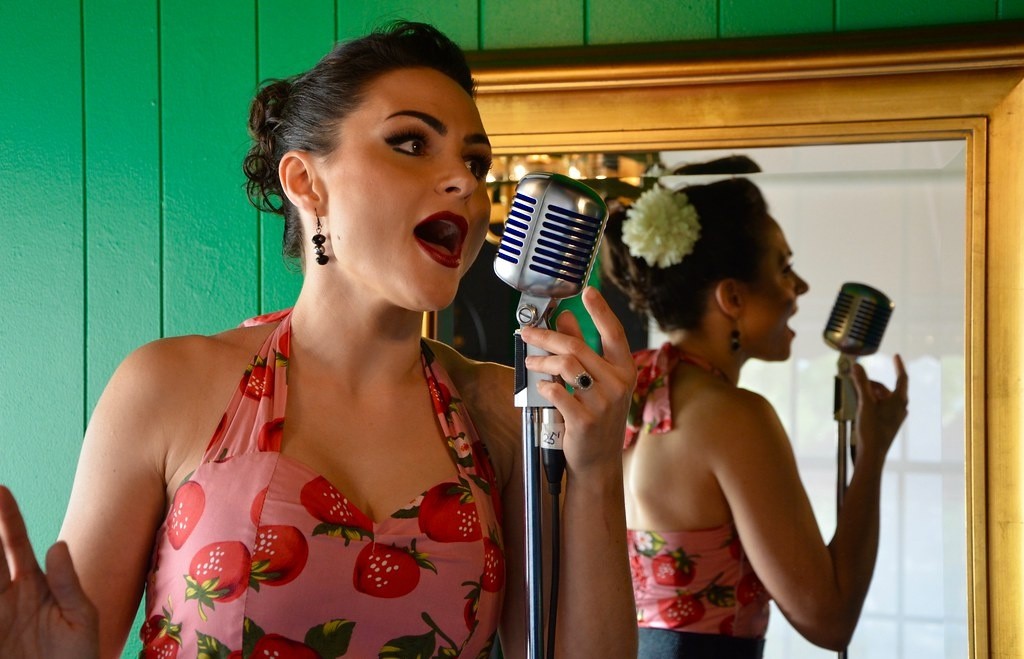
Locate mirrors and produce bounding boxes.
[422,17,1024,659]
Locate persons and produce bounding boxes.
[0,21,638,659]
[622,177,908,659]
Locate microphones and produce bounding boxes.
[492,171,610,330]
[822,282,895,366]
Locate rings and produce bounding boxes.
[572,371,593,391]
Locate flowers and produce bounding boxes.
[622,180,702,271]
